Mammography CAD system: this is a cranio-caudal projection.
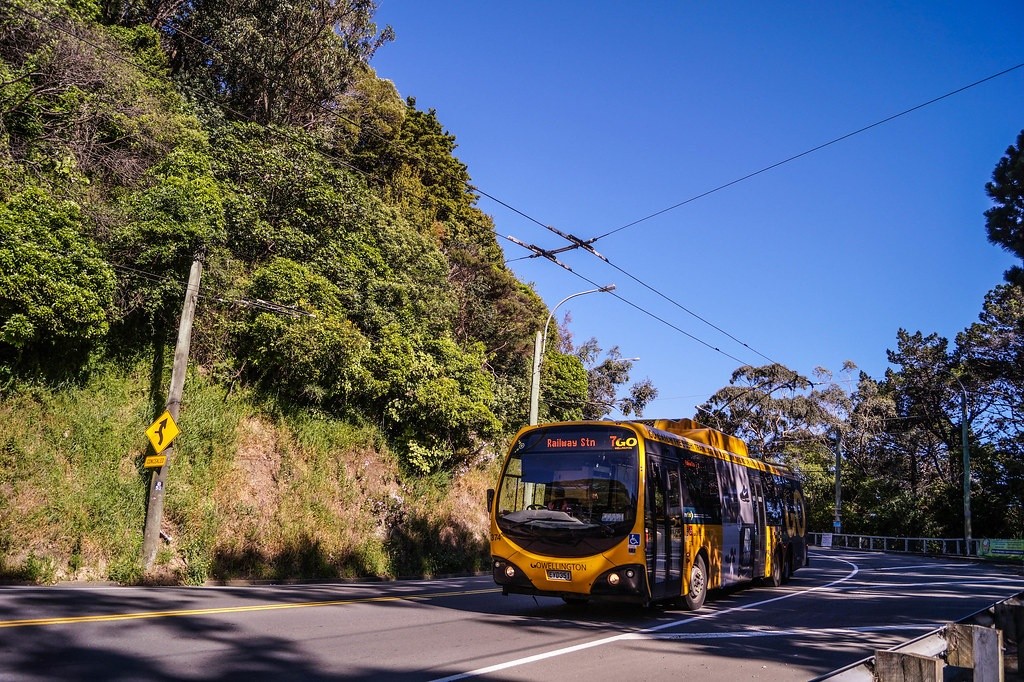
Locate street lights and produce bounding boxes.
[924,363,975,559]
[521,282,618,510]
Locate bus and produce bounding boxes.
[486,418,809,610]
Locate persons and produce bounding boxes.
[547,487,573,512]
